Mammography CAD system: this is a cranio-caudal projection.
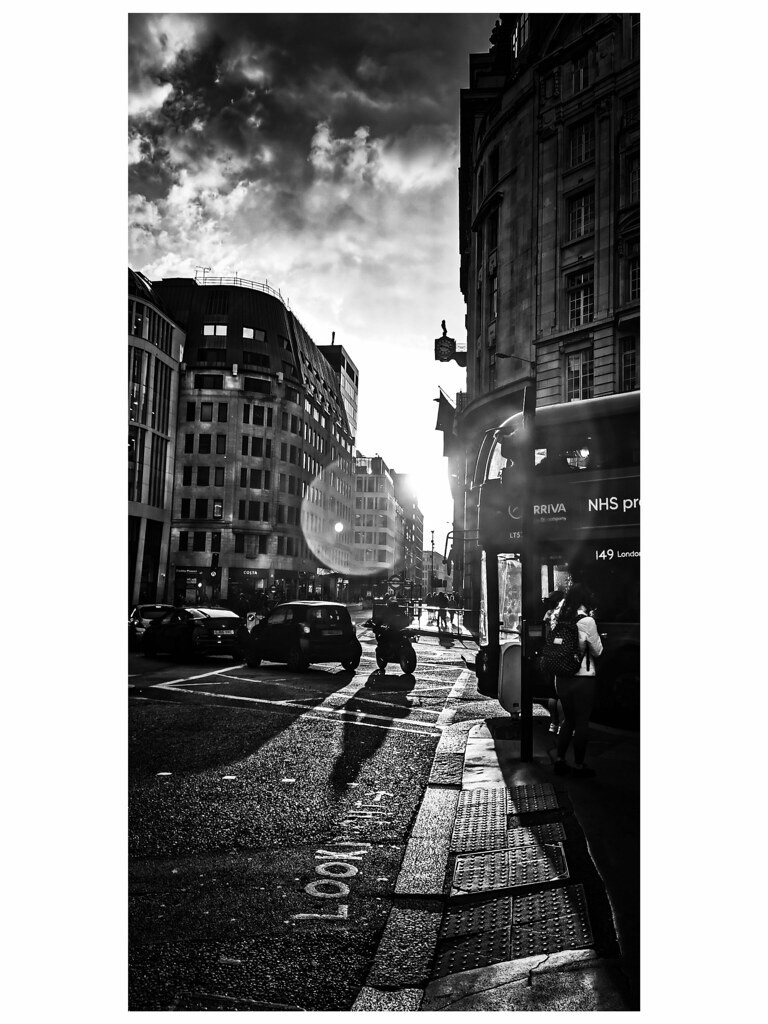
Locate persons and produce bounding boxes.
[379,597,411,659]
[543,593,577,736]
[552,584,604,779]
[426,591,464,626]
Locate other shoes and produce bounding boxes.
[555,760,596,777]
[549,724,576,736]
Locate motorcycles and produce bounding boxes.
[365,622,417,674]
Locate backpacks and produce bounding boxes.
[541,615,591,674]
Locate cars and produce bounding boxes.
[130,604,176,643]
[247,599,359,672]
[143,608,249,661]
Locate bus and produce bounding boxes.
[443,388,640,734]
